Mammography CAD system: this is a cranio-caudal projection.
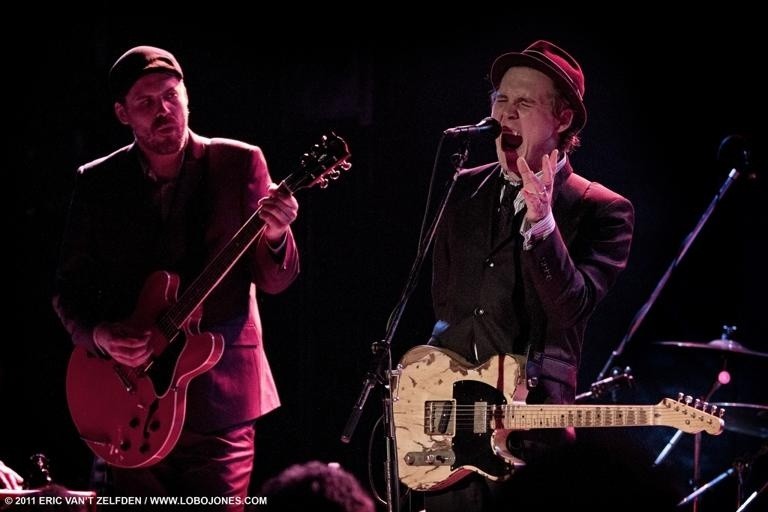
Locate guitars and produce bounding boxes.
[66,129,352,468]
[392,345,725,492]
[368,366,636,506]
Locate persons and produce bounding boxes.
[52,44,301,512]
[423,40,635,512]
[249,460,377,512]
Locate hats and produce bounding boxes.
[109,45,184,99]
[491,40,588,134]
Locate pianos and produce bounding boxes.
[697,402,768,448]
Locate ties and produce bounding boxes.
[494,180,523,247]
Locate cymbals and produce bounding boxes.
[662,340,768,356]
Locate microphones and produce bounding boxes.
[443,116,502,141]
